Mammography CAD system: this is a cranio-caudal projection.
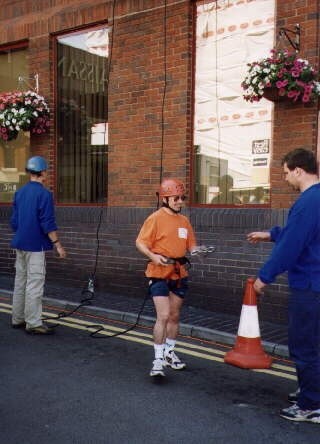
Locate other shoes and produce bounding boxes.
[12,318,55,336]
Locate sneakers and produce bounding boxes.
[165,350,186,370]
[149,359,164,376]
[280,388,320,423]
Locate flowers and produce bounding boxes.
[240,46,320,103]
[0,89,51,142]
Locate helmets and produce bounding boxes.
[157,179,186,197]
[25,156,48,173]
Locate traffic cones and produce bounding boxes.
[221,277,273,369]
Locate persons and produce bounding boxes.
[9,156,66,335]
[212,175,241,203]
[247,148,320,423]
[251,187,264,204]
[136,179,207,376]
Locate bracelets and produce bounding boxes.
[53,240,59,243]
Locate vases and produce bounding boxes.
[261,85,292,103]
